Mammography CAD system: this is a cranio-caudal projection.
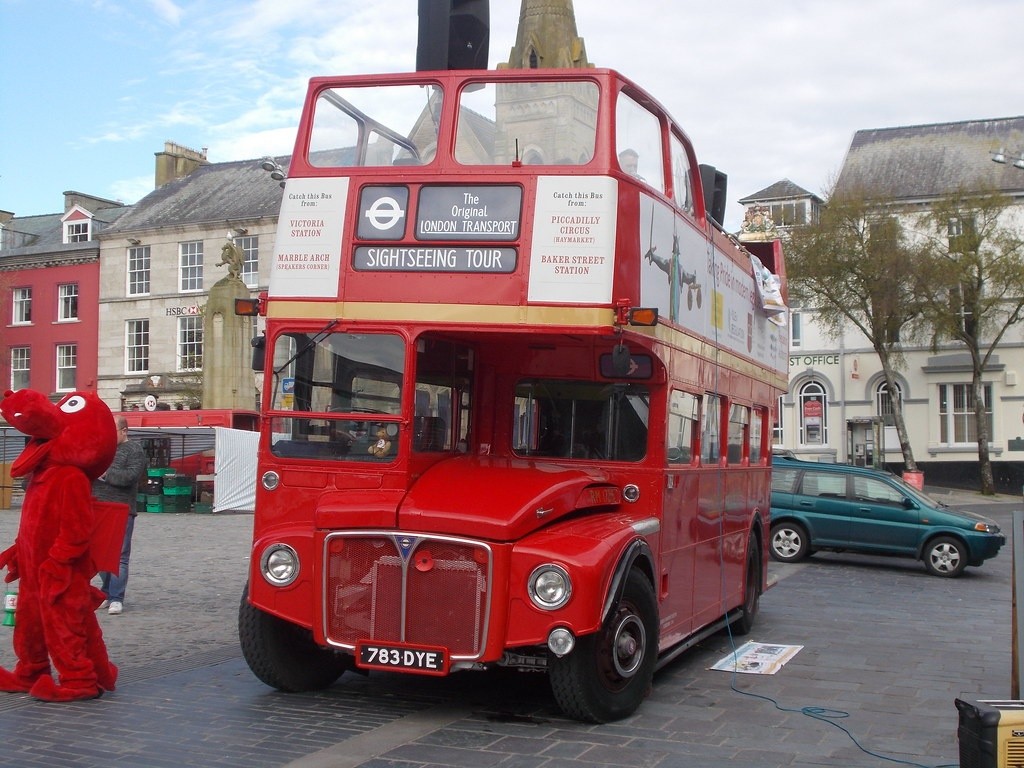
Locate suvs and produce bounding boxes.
[769,459,1007,579]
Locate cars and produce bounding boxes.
[725,444,800,482]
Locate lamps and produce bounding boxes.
[233,229,248,233]
[127,238,141,244]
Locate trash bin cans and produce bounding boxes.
[818,455,836,465]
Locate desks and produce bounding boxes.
[196,475,214,502]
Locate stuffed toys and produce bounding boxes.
[367,431,392,460]
[0,389,118,702]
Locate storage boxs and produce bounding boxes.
[136,468,212,513]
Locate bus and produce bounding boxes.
[106,410,281,491]
[233,68,793,731]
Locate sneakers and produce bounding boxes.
[108,601,124,613]
[98,599,110,608]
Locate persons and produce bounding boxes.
[93,415,145,614]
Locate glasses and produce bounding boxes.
[117,427,125,431]
